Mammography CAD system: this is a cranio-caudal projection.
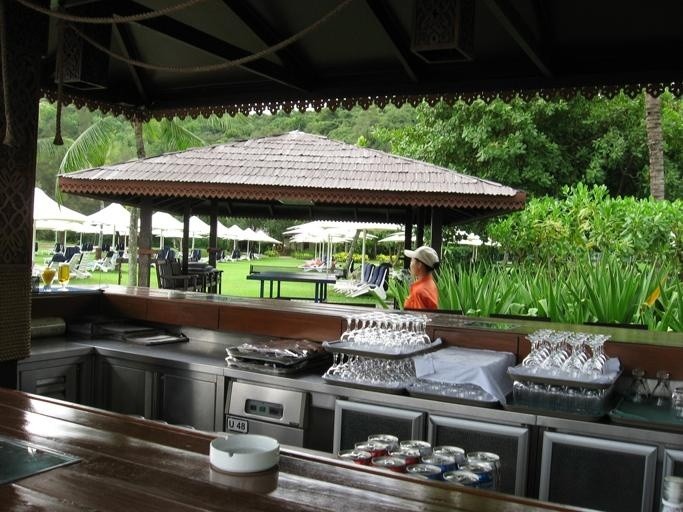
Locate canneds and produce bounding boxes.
[338,434,501,492]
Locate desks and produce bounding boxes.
[244,264,336,304]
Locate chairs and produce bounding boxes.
[148,245,223,296]
[217,248,261,263]
[32,241,125,282]
[331,261,391,300]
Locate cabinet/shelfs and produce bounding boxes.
[94,357,226,438]
[17,359,91,406]
[332,400,657,509]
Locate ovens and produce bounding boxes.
[223,377,311,447]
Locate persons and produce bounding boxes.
[401,247,441,312]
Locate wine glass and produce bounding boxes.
[626,368,649,404]
[326,352,417,387]
[58,263,70,292]
[340,311,433,354]
[650,370,672,409]
[521,328,613,377]
[38,268,57,292]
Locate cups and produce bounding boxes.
[671,386,683,424]
[512,375,626,417]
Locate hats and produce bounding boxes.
[403,246,440,269]
